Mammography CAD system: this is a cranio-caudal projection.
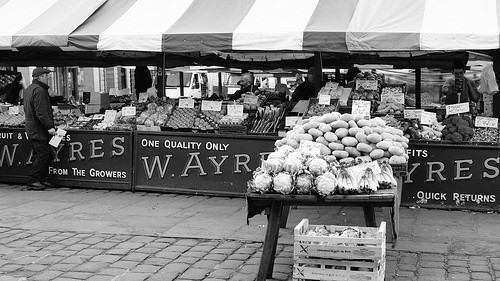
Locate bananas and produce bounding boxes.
[337,158,397,193]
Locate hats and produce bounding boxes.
[32,68,51,77]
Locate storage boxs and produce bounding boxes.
[293,218,387,281]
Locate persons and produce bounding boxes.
[344,67,360,81]
[229,73,259,100]
[291,66,323,102]
[442,67,481,115]
[23,68,57,190]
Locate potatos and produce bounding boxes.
[274,111,410,165]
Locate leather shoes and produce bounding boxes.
[41,182,51,188]
[27,182,45,190]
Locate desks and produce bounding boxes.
[246,178,402,281]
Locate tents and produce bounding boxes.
[1,0,500,109]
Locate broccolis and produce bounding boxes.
[247,144,338,198]
[304,225,378,246]
